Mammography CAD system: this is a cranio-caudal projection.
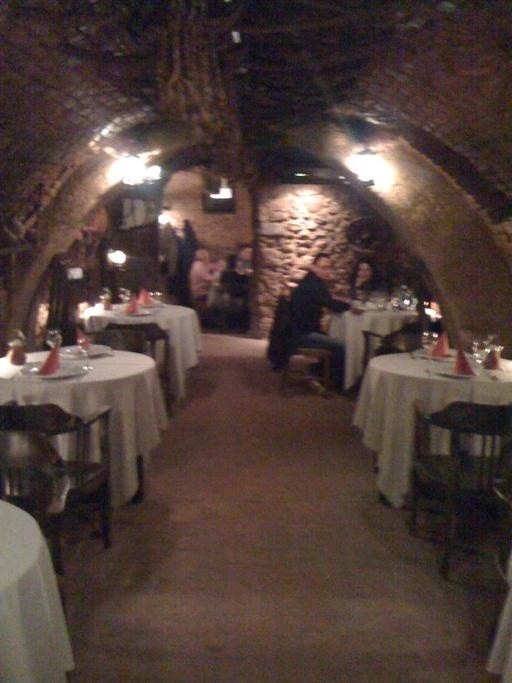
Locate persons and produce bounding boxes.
[187,244,255,335]
[349,254,386,299]
[290,252,365,397]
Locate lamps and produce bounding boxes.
[209,176,233,200]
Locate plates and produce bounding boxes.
[19,345,111,378]
[120,309,150,315]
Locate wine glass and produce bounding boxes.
[472,334,504,369]
[421,332,439,360]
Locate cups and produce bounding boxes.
[5,328,63,348]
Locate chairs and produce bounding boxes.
[77,330,147,355]
[0,419,71,575]
[385,323,439,375]
[409,398,512,579]
[172,274,224,328]
[273,293,331,400]
[363,331,403,370]
[105,324,176,416]
[0,400,113,549]
